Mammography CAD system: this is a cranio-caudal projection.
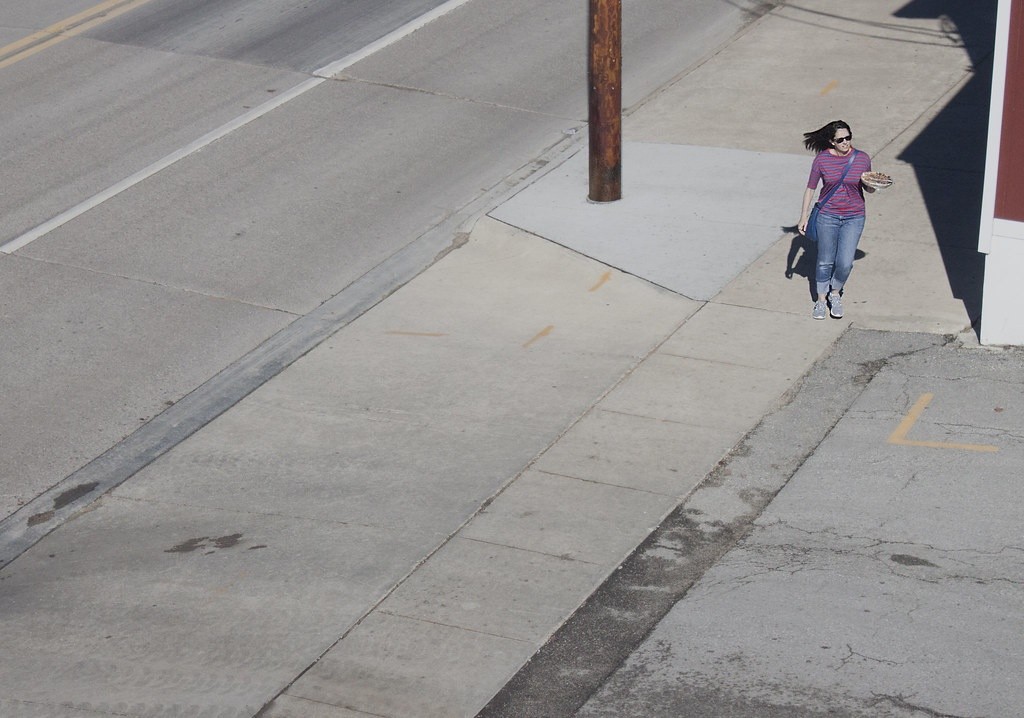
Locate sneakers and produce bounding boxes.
[828,292,844,317]
[812,300,827,319]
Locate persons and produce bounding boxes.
[798,120,876,320]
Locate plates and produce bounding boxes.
[861,172,892,188]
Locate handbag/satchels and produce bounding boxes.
[805,204,821,243]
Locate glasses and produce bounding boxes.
[832,134,852,144]
